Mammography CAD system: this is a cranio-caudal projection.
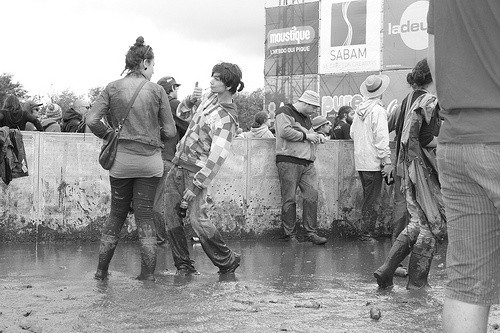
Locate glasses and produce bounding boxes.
[32,108,39,111]
[145,45,152,55]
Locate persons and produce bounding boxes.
[237,108,277,140]
[374,59,447,291]
[350,76,395,244]
[427,0,500,333]
[154,76,188,248]
[274,90,327,244]
[85,37,178,281]
[165,63,244,276]
[311,106,355,141]
[0,93,111,134]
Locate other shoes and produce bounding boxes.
[308,235,327,245]
[286,234,296,241]
[177,260,195,272]
[220,255,242,272]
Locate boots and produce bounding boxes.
[406,245,435,290]
[135,236,158,282]
[374,234,416,287]
[96,232,118,278]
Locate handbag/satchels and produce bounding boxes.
[98,129,120,169]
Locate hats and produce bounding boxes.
[46,104,62,120]
[312,115,328,130]
[298,90,321,107]
[31,103,43,107]
[157,77,182,92]
[360,75,390,98]
